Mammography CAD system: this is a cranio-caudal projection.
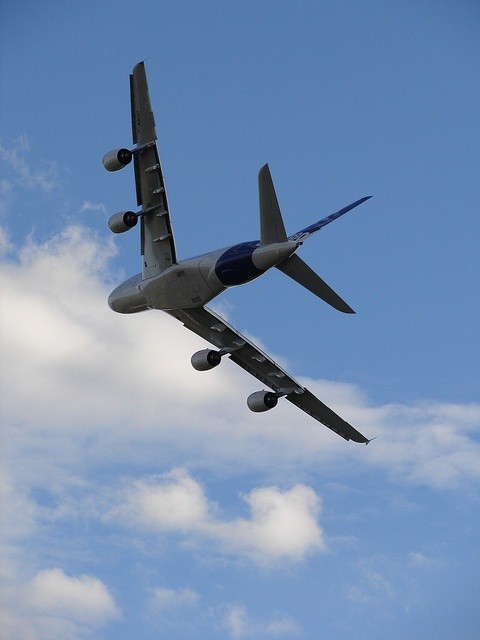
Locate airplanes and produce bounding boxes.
[102,61,378,447]
